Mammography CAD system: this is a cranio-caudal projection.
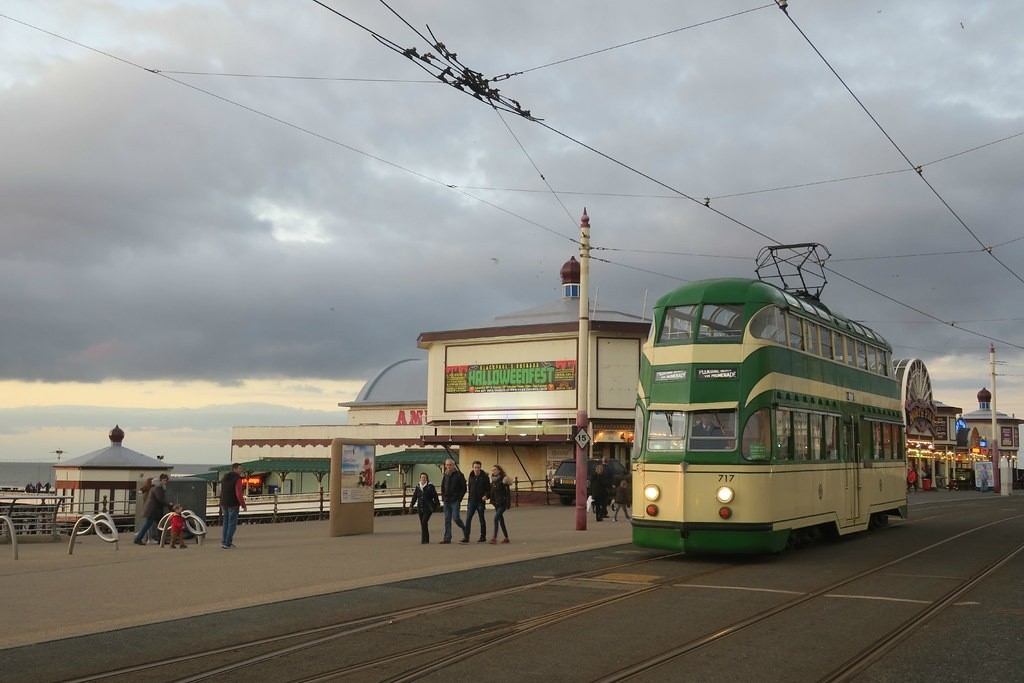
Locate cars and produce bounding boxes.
[550,457,630,505]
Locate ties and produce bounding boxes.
[704,427,708,431]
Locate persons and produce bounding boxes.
[407,471,442,543]
[611,480,633,522]
[587,464,611,521]
[169,504,191,549]
[35,480,44,492]
[438,458,467,544]
[375,479,382,489]
[44,480,51,492]
[905,467,918,493]
[380,479,389,489]
[132,474,174,545]
[457,460,490,543]
[218,462,247,549]
[687,412,731,450]
[133,476,159,545]
[481,464,512,544]
[595,455,616,518]
[980,464,989,491]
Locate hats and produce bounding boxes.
[363,457,372,469]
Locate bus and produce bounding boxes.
[629,242,908,559]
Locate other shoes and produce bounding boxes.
[170,545,176,548]
[223,543,236,549]
[627,518,632,521]
[457,539,470,545]
[222,542,226,547]
[180,544,187,548]
[147,539,159,545]
[501,538,510,545]
[158,540,170,544]
[489,538,497,544]
[602,516,609,518]
[477,538,487,544]
[439,540,451,544]
[134,540,146,545]
[613,519,618,521]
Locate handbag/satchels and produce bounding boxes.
[586,495,595,513]
